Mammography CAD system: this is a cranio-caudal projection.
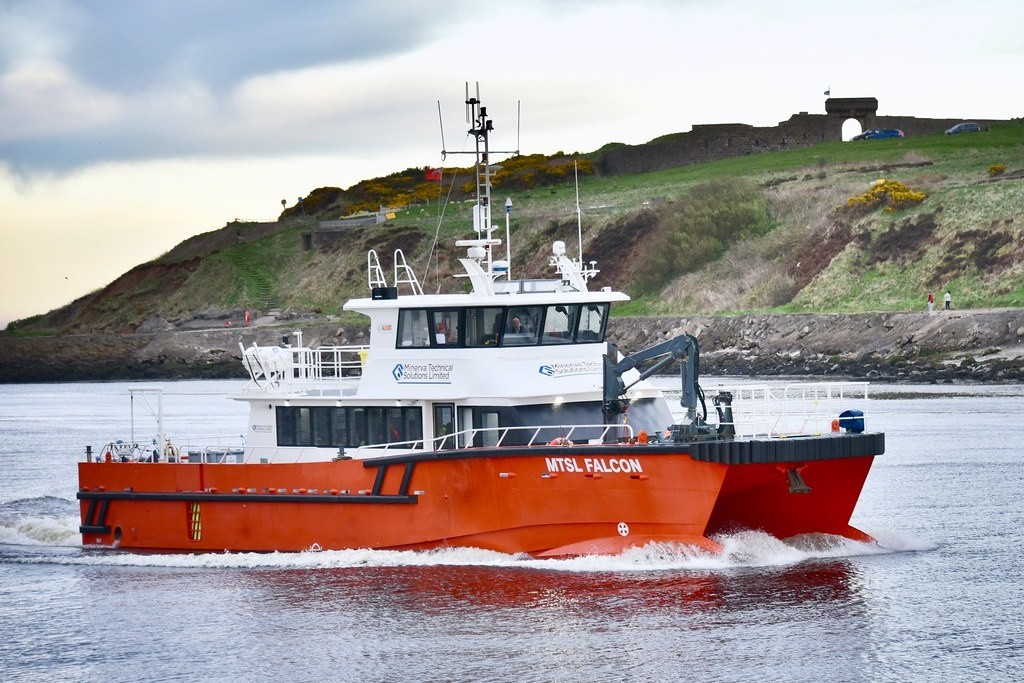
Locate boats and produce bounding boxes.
[76,80,886,560]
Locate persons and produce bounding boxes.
[928,290,934,317]
[509,318,524,333]
[436,323,449,342]
[245,311,250,326]
[944,291,951,309]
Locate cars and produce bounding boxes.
[853,129,905,140]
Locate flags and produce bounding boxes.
[824,91,829,95]
[425,168,442,181]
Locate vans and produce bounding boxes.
[946,123,981,134]
